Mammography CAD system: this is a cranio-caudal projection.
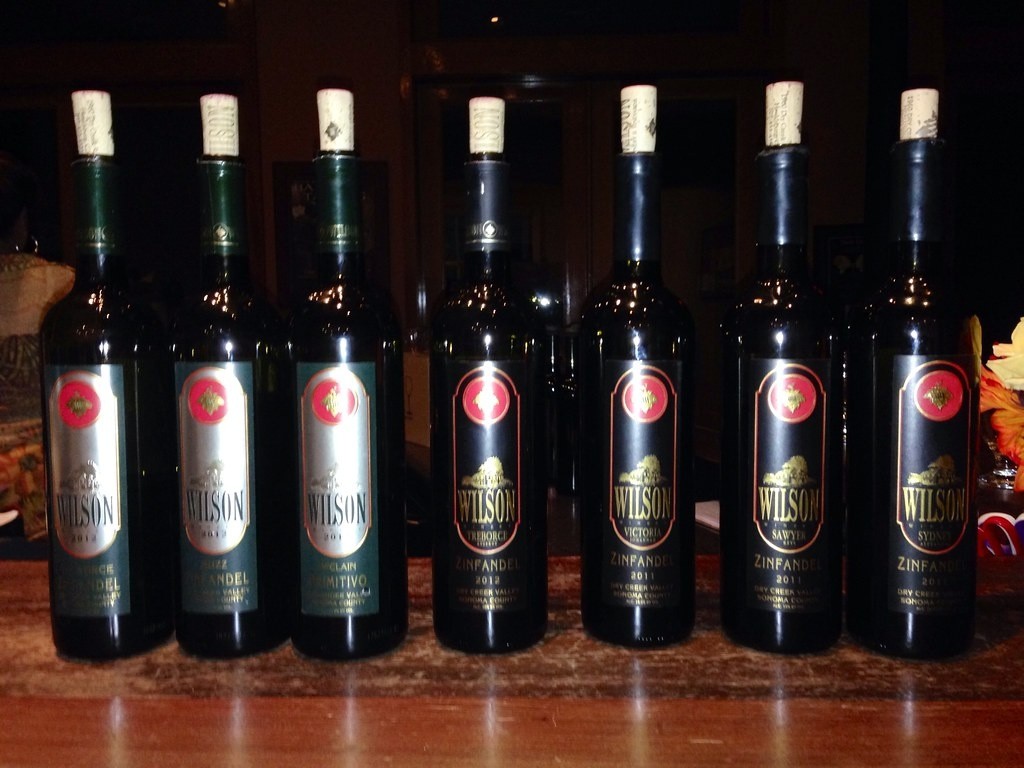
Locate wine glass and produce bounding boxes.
[978,417,1018,492]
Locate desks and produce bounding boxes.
[0,558,1024,768]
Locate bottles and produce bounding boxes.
[723,80,845,654]
[576,86,701,648]
[39,89,174,664]
[170,94,293,658]
[431,96,548,656]
[870,88,979,664]
[280,87,408,661]
